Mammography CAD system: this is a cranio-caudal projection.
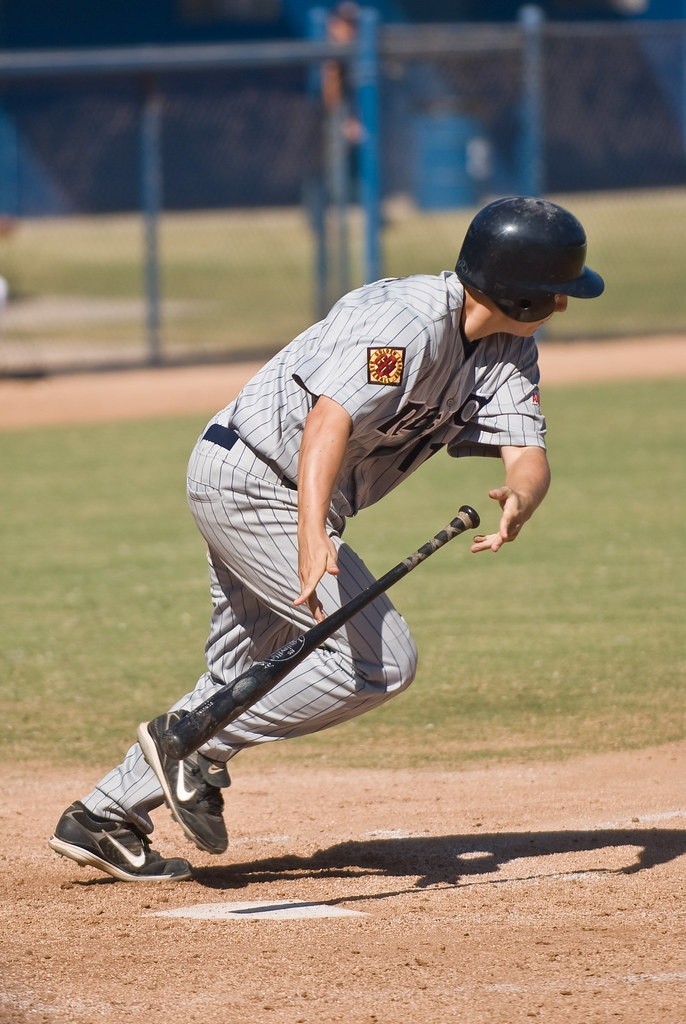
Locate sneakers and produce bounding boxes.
[48,801,193,881]
[137,710,231,854]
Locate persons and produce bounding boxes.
[302,1,387,228]
[47,196,605,882]
[0,118,24,303]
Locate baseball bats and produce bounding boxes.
[157,501,484,765]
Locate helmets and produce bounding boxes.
[455,196,604,321]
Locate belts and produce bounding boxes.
[203,422,238,452]
